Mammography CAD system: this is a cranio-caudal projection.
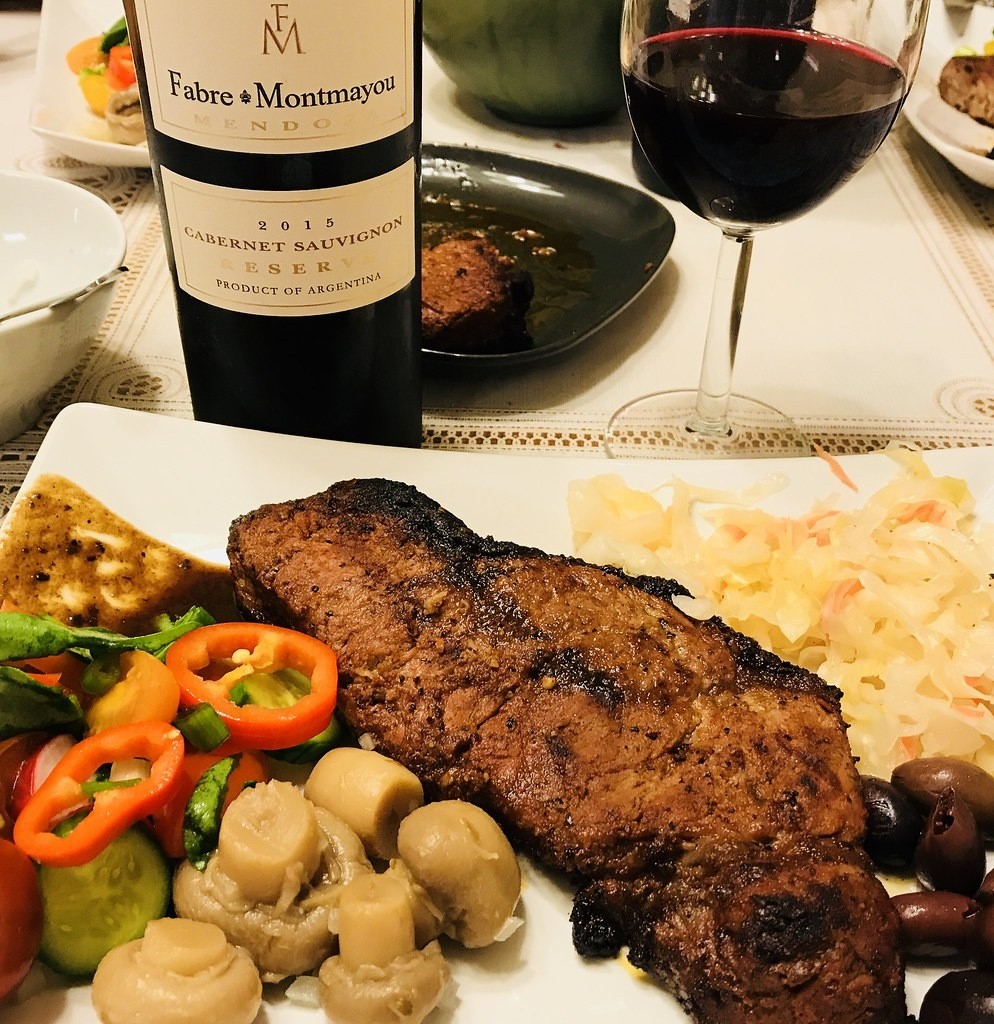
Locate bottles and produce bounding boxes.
[630,2,817,205]
[120,0,423,449]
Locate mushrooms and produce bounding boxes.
[0,477,526,1022]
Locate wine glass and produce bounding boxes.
[606,1,929,460]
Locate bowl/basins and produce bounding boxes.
[421,0,626,130]
[0,173,129,447]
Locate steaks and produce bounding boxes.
[938,54,994,128]
[227,477,909,1024]
[418,221,532,346]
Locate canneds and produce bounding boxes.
[128,1,425,451]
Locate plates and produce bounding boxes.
[418,140,677,375]
[900,2,994,190]
[1,401,993,1023]
[27,0,150,169]
